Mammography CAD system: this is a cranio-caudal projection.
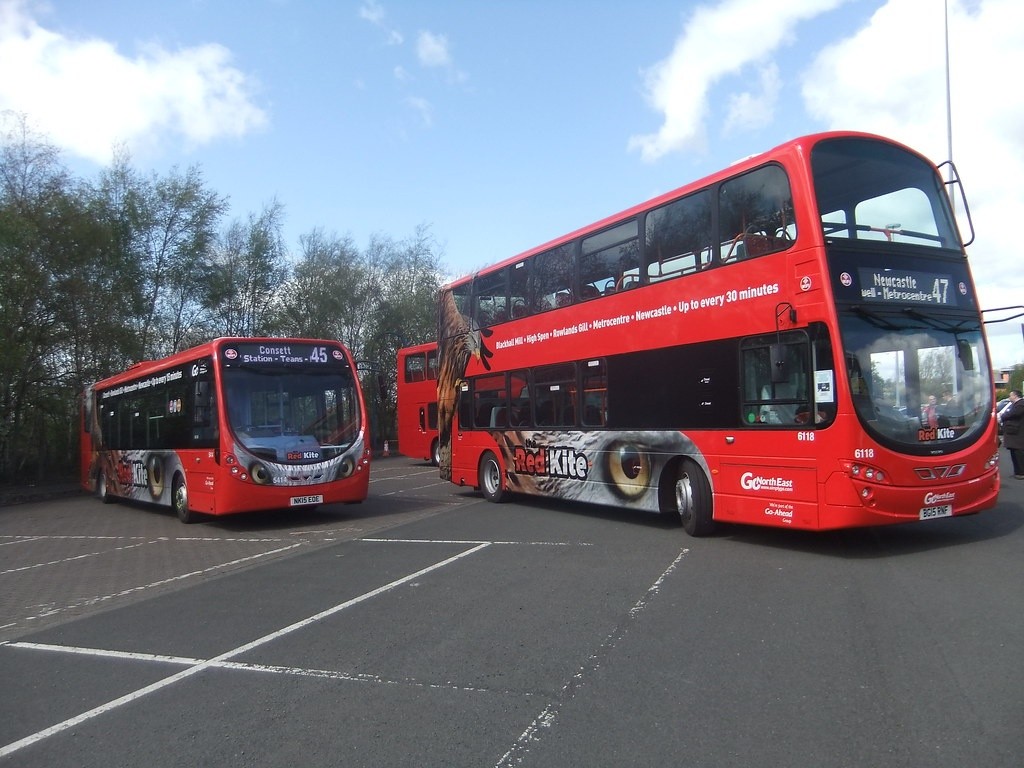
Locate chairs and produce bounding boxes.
[743,225,793,258]
[478,280,617,327]
[459,402,603,427]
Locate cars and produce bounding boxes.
[899,399,1012,433]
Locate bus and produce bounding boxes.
[80,336,388,525]
[437,130,1024,537]
[398,341,607,466]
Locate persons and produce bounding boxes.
[936,390,958,428]
[997,390,1024,479]
[921,395,939,428]
[758,378,826,423]
[511,404,529,425]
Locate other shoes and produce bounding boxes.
[1009,475,1024,480]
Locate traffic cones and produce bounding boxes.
[383,440,391,457]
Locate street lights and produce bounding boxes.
[886,222,901,408]
